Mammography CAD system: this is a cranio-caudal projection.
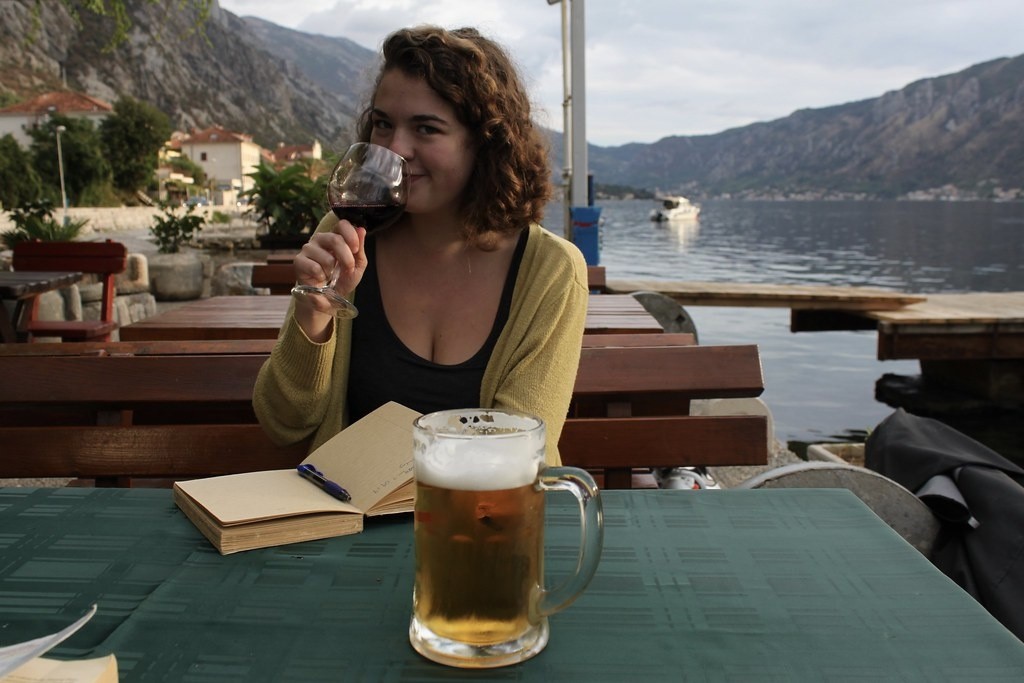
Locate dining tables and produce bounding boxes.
[0,486,1023,683]
[119,294,664,341]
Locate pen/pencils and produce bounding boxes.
[295,463,354,504]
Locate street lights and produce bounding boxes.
[56,125,68,208]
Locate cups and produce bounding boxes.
[408,410,604,670]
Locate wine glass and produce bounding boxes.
[290,141,411,319]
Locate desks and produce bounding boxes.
[1,267,83,336]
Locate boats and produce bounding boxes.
[649,196,701,222]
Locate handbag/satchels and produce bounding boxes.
[859,405,1023,647]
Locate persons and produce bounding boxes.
[252,25,600,491]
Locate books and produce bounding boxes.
[5,605,120,683]
[173,401,423,555]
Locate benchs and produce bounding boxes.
[0,333,769,491]
[9,240,127,342]
[249,263,607,296]
